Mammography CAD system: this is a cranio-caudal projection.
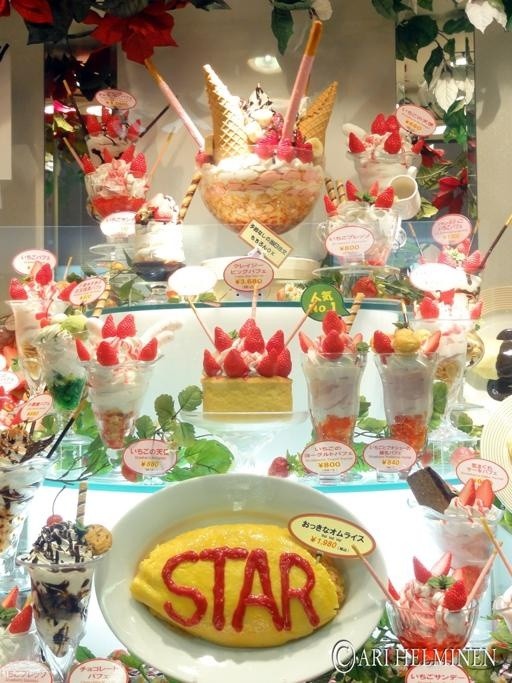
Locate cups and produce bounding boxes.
[387,166,422,220]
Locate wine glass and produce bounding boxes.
[407,498,503,644]
[0,300,159,683]
[84,131,185,306]
[303,205,491,470]
[180,410,308,474]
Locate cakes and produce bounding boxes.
[189,284,320,423]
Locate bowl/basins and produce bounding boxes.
[194,143,325,236]
[346,151,421,195]
[385,595,479,661]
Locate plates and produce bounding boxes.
[95,474,390,683]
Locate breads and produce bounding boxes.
[129,522,346,648]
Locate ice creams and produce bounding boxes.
[0,80,190,683]
[298,112,512,683]
[144,18,340,261]
